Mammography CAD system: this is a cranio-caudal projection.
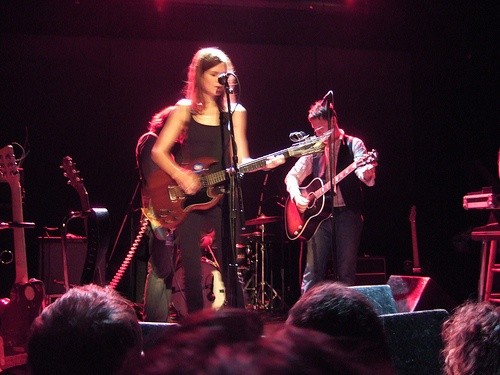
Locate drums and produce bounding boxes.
[236,244,253,271]
[172,257,226,316]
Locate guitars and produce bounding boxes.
[403,205,427,277]
[140,137,324,229]
[284,149,378,242]
[60,155,89,235]
[0,146,45,372]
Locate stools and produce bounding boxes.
[471,231,500,305]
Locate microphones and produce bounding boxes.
[217,72,231,85]
[317,91,332,111]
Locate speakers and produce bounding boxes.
[349,284,397,313]
[388,275,454,312]
[380,308,453,375]
[354,273,387,285]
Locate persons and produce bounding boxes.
[286,100,376,296]
[151,47,286,313]
[27,282,500,375]
[136,107,188,322]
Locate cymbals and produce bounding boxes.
[240,232,278,237]
[244,216,282,226]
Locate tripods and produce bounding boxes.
[241,225,289,309]
